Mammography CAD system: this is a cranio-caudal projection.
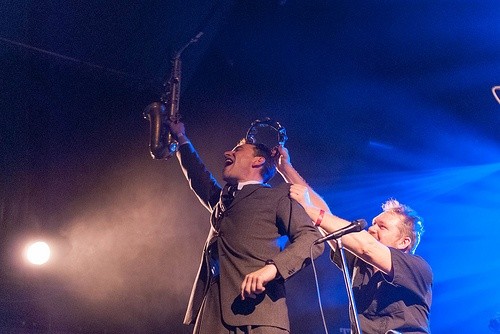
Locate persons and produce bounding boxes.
[271,146,433,334]
[166,121,325,334]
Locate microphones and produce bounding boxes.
[313,219,367,244]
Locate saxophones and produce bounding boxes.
[143,30,205,159]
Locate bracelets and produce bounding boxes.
[315,209,325,227]
[174,133,185,139]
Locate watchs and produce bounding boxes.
[265,260,281,280]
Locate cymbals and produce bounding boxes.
[246,117,288,161]
